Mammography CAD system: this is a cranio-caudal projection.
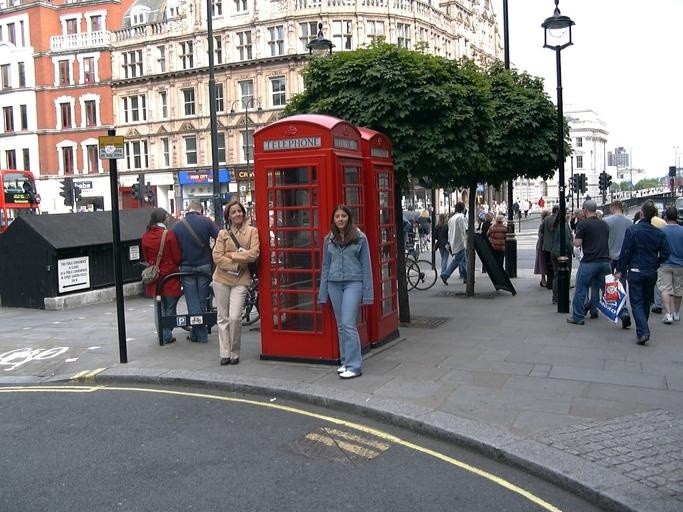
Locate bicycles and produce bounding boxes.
[403,231,438,294]
[239,260,286,328]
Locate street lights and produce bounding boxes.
[672,146,680,169]
[307,22,336,57]
[229,95,264,218]
[541,0,583,310]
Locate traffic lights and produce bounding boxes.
[145,183,155,202]
[74,186,82,202]
[131,183,141,200]
[565,169,614,197]
[60,176,73,208]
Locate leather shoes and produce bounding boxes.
[621,317,629,328]
[463,280,475,283]
[567,304,601,324]
[439,274,448,285]
[635,333,650,343]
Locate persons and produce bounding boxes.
[209,199,260,366]
[513,196,532,218]
[171,202,219,344]
[532,196,682,346]
[316,203,375,379]
[402,195,509,286]
[141,208,182,344]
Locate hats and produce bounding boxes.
[494,215,506,221]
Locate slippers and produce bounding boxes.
[539,279,548,287]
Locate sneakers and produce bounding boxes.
[230,357,239,365]
[651,305,679,323]
[186,335,198,343]
[166,337,176,345]
[336,364,346,373]
[220,358,230,365]
[339,370,363,379]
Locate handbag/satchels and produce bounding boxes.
[445,243,455,257]
[596,280,628,324]
[433,240,440,252]
[141,266,159,284]
[605,274,620,302]
[248,259,259,276]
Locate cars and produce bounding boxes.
[674,196,683,224]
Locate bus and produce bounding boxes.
[0,170,42,236]
